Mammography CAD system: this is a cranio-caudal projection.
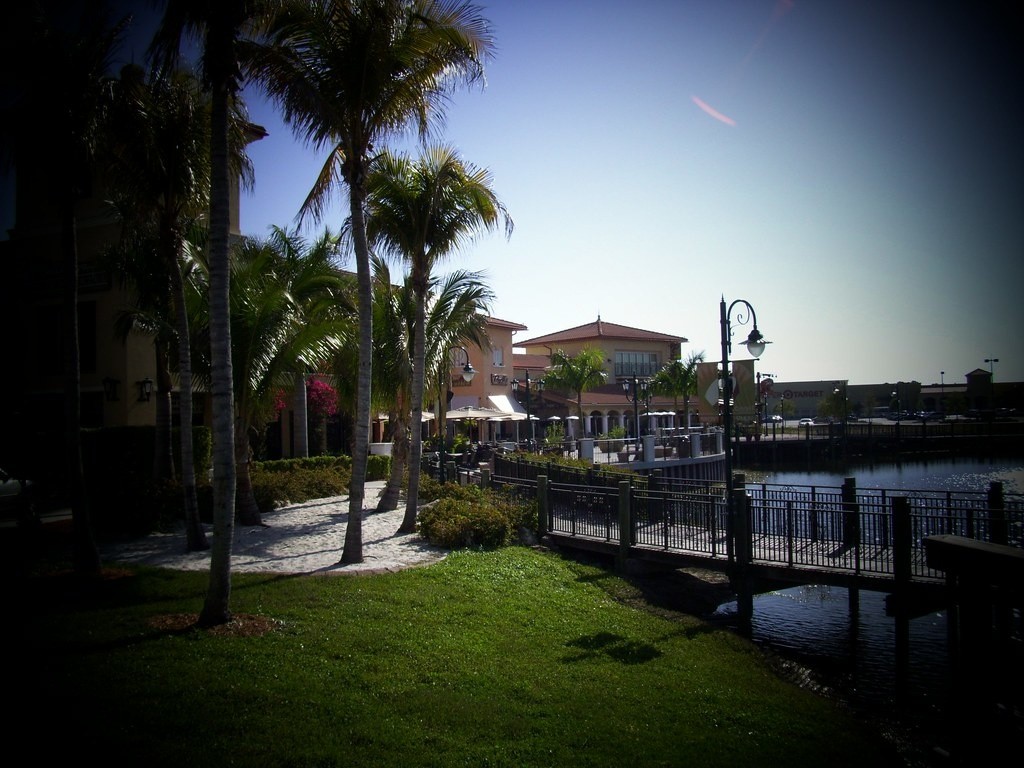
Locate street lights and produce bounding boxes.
[780,395,785,434]
[984,359,999,422]
[833,386,840,437]
[438,346,480,485]
[720,294,773,562]
[621,370,648,459]
[510,369,545,453]
[764,392,768,436]
[892,384,901,424]
[941,372,944,423]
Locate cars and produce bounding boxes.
[0,469,37,520]
[888,411,942,422]
[965,408,1016,417]
[799,418,815,427]
[767,414,782,423]
[847,415,857,421]
[812,416,833,424]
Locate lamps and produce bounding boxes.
[141,377,154,401]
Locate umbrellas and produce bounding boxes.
[374,403,539,452]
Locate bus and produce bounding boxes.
[862,406,890,418]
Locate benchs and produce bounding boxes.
[618,451,641,463]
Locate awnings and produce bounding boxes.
[487,395,528,414]
